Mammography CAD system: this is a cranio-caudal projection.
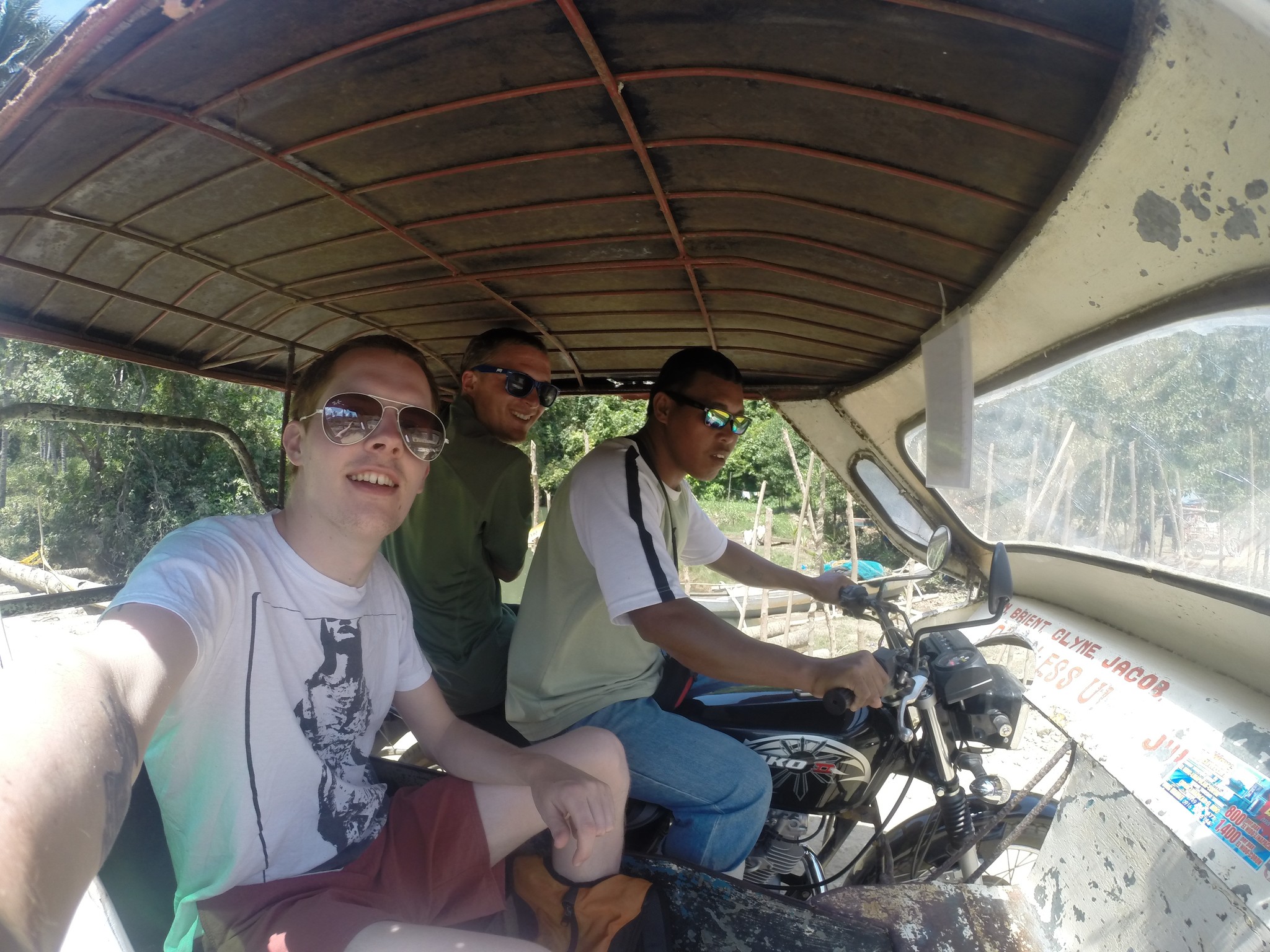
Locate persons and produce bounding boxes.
[379,325,554,734]
[503,348,888,875]
[0,337,630,952]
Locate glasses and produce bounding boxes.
[300,390,451,462]
[670,393,752,435]
[470,364,561,408]
